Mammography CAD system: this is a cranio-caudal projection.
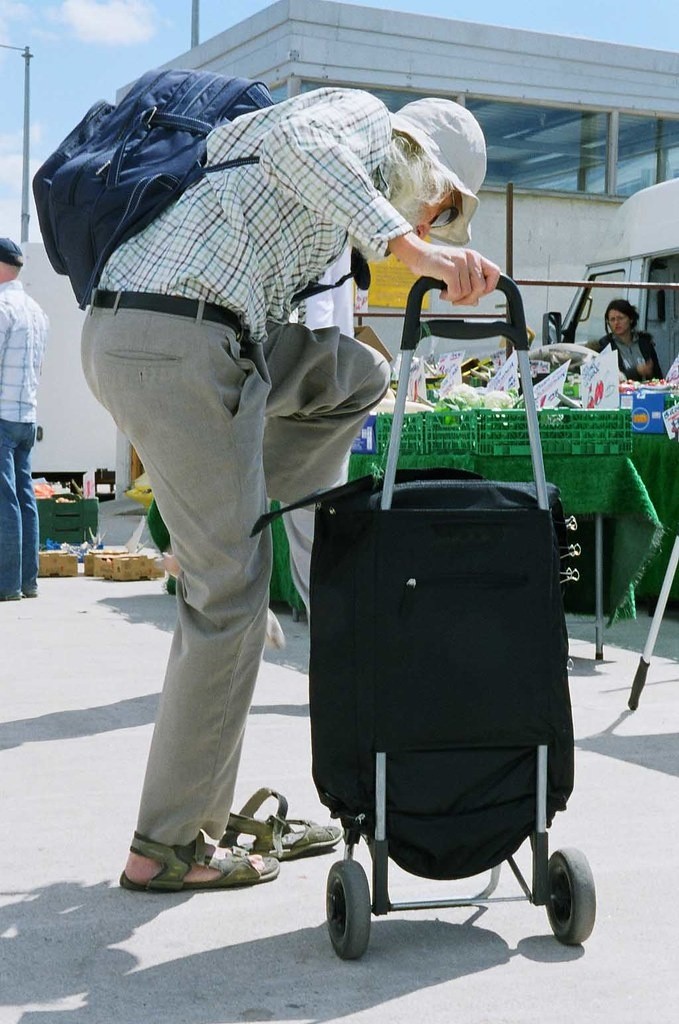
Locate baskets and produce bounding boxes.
[376,407,633,456]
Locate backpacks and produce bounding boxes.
[32,72,275,311]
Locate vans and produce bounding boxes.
[538,177,679,383]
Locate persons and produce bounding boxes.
[599,300,664,381]
[0,237,49,600]
[80,89,501,892]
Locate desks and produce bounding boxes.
[268,455,664,662]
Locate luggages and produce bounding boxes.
[303,271,597,961]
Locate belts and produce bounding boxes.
[93,289,243,341]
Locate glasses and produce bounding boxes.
[430,191,459,228]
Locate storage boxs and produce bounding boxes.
[40,542,167,581]
[35,491,100,549]
[350,407,631,456]
[621,388,679,434]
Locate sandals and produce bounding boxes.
[120,830,280,893]
[219,788,343,862]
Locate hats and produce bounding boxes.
[390,98,487,247]
[0,237,23,266]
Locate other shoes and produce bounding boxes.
[0,591,37,601]
